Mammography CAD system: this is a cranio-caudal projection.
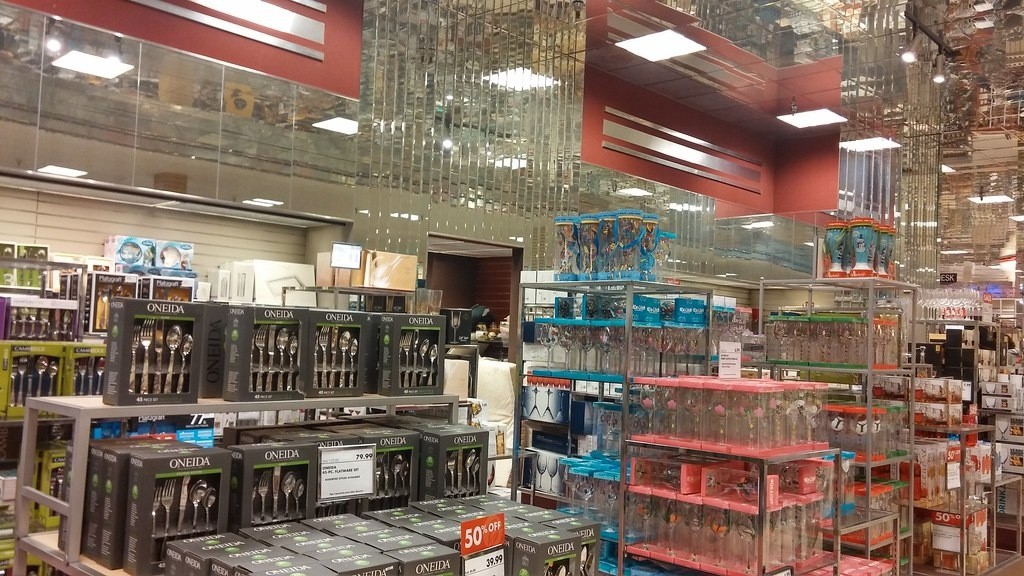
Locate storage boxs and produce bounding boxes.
[0,243,604,576]
[912,348,1024,576]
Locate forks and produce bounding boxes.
[249,324,268,392]
[10,354,24,408]
[127,318,156,395]
[374,454,384,496]
[249,469,272,523]
[312,325,330,388]
[398,330,414,387]
[442,450,459,494]
[73,356,88,395]
[148,477,177,561]
[49,468,64,498]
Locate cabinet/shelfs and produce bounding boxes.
[913,319,1024,576]
[515,277,918,576]
[13,389,459,576]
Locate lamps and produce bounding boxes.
[901,34,923,64]
[46,34,63,51]
[437,126,452,148]
[932,54,945,82]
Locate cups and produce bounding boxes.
[413,288,443,315]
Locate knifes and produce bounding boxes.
[265,325,277,392]
[26,353,35,401]
[383,450,391,496]
[87,356,95,395]
[177,475,192,533]
[456,449,463,492]
[409,328,421,387]
[153,319,162,394]
[328,326,339,388]
[579,546,587,576]
[270,465,281,519]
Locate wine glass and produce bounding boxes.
[449,311,463,345]
[536,213,1024,576]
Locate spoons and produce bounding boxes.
[35,354,58,397]
[164,325,193,393]
[95,357,106,394]
[189,479,217,536]
[556,565,567,576]
[338,330,359,387]
[417,340,439,386]
[9,282,133,338]
[276,326,298,392]
[280,471,305,517]
[465,449,481,492]
[389,453,409,493]
